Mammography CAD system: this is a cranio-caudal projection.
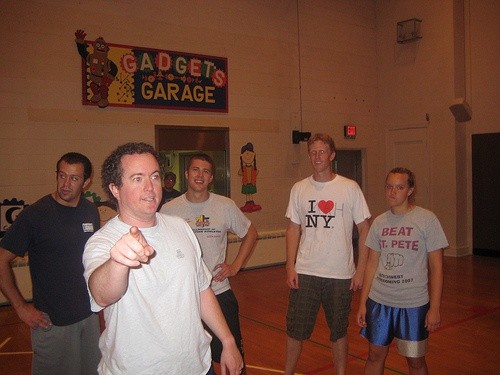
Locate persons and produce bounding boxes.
[356,168,450,375]
[158,153,258,375]
[0,152,103,375]
[81,143,245,375]
[156,172,183,212]
[282,133,372,375]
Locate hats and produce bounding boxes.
[165,171,176,180]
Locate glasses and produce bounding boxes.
[166,179,174,181]
